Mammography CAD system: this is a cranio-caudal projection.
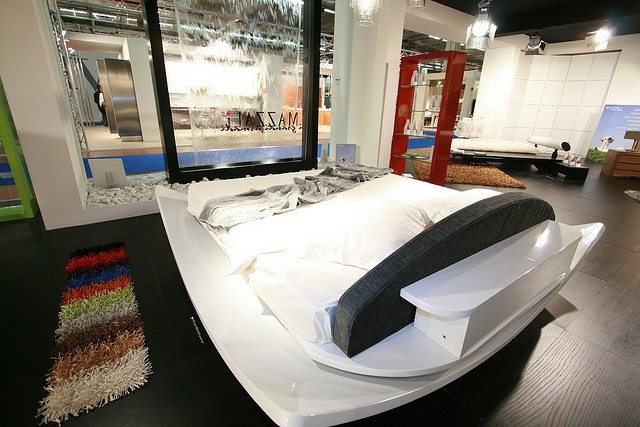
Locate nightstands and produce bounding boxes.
[549,162,588,181]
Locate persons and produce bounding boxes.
[94,85,108,128]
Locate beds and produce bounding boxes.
[451,136,570,161]
[188,164,582,377]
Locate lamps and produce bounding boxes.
[466,1,497,51]
[521,31,548,55]
[624,130,640,151]
[350,1,384,26]
[585,24,615,52]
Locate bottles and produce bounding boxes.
[411,70,419,87]
[568,155,576,166]
[562,152,570,164]
[576,157,581,167]
[435,94,442,110]
[428,95,436,110]
[404,118,411,136]
[417,68,427,85]
[417,120,424,136]
[409,119,417,134]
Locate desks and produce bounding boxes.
[600,148,640,180]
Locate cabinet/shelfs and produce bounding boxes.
[390,50,467,186]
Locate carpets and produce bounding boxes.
[36,241,151,426]
[413,158,526,190]
[624,190,640,204]
